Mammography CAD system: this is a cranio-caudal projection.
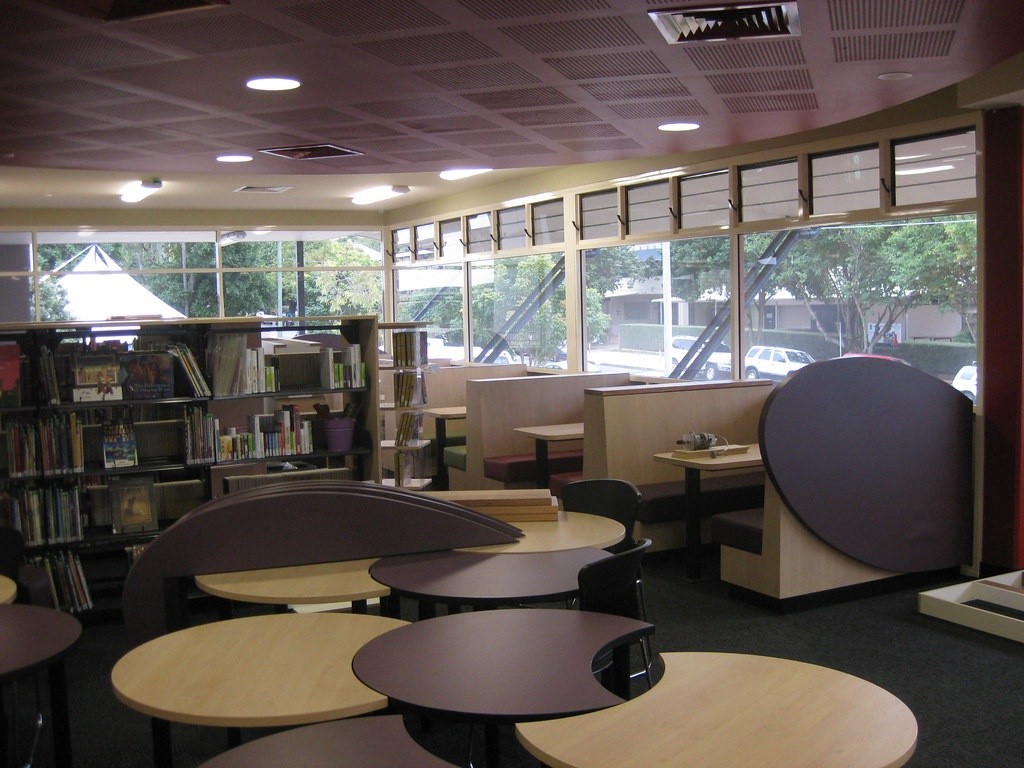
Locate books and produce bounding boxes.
[182,404,313,463]
[34,346,61,406]
[153,341,212,398]
[96,340,140,353]
[0,405,181,477]
[0,476,149,611]
[207,332,366,397]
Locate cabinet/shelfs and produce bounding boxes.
[379,320,435,491]
[0,316,381,628]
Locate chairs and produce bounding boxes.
[573,537,654,691]
[516,476,643,608]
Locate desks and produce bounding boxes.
[119,478,627,622]
[0,478,83,768]
[113,613,919,768]
[513,423,585,490]
[654,443,768,582]
[421,406,466,491]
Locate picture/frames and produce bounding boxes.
[110,476,159,535]
[69,348,122,389]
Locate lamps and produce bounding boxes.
[352,185,410,206]
[439,168,495,181]
[120,178,164,204]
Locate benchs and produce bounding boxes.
[377,352,980,618]
